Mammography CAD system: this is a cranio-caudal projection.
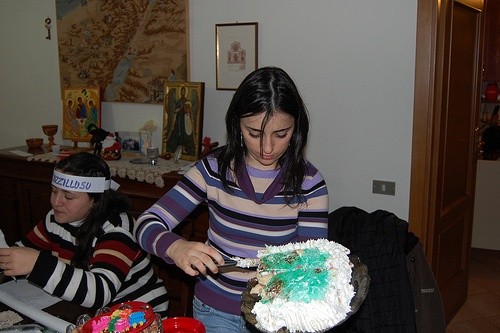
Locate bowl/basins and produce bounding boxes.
[26,138,43,149]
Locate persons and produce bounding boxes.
[0,152,171,320]
[132,67,329,333]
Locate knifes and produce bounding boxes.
[191,258,259,269]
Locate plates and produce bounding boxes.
[161,317,206,333]
[130,158,150,163]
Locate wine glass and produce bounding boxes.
[147,147,159,166]
[42,124,58,148]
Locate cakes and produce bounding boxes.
[251,238,357,333]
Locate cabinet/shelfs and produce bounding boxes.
[0,143,209,318]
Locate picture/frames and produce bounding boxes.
[63,85,101,142]
[215,21,259,91]
[161,81,205,161]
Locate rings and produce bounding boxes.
[5,262,8,270]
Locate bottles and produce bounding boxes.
[485,82,498,102]
[482,105,488,123]
